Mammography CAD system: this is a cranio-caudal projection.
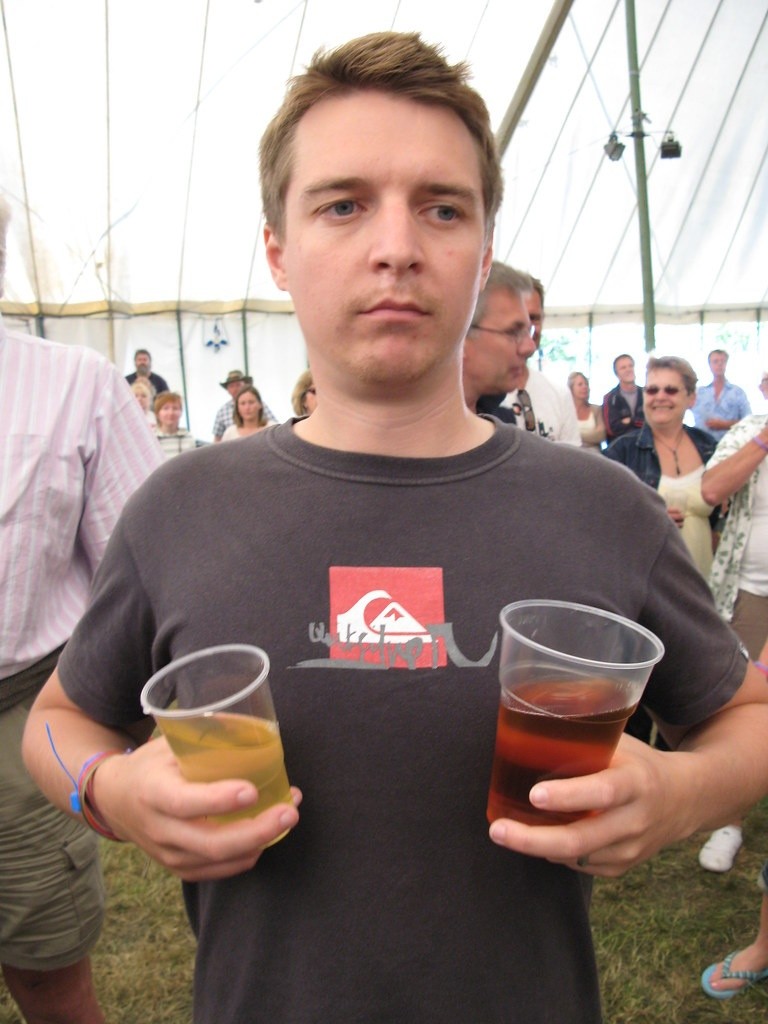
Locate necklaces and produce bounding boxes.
[652,428,684,475]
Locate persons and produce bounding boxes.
[690,349,752,443]
[0,196,172,1023]
[498,270,583,452]
[125,349,319,461]
[701,372,768,1000]
[601,355,733,753]
[20,28,767,1024]
[565,372,607,453]
[462,259,537,428]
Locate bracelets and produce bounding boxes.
[41,719,134,843]
[752,436,768,452]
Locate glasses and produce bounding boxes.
[470,324,536,344]
[302,388,315,397]
[642,385,688,395]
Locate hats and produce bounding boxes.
[219,370,252,389]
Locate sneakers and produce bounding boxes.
[698,824,743,872]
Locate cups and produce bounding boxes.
[486,598,665,841]
[139,644,293,851]
[666,490,688,528]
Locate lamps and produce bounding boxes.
[604,132,632,161]
[647,130,681,158]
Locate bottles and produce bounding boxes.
[539,422,546,436]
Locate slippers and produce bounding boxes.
[700,949,768,999]
